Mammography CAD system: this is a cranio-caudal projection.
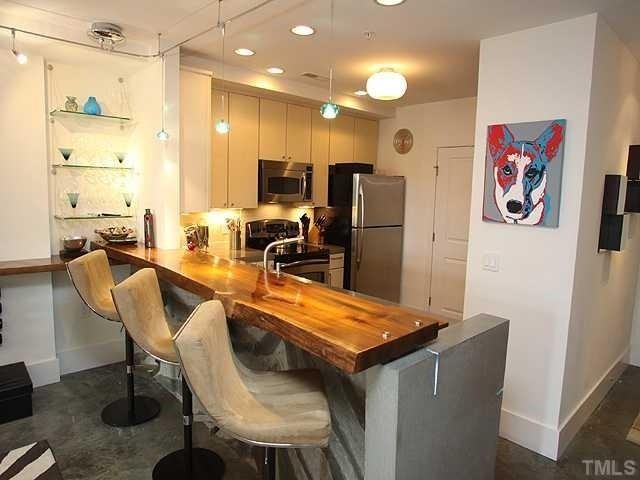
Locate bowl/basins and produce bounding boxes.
[63,236,87,249]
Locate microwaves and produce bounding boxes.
[258,159,314,203]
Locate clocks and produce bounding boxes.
[391,127,413,155]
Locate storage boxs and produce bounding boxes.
[0,362,34,424]
[0,438,64,480]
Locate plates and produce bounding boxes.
[94,228,133,242]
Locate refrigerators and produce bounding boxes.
[328,162,405,308]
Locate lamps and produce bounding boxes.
[7,28,30,66]
[320,1,342,120]
[214,22,231,135]
[157,52,171,142]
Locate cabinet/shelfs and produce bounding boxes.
[260,99,312,165]
[48,105,135,221]
[355,117,379,164]
[310,108,330,207]
[328,111,355,169]
[209,86,260,209]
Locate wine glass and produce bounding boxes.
[198,225,209,253]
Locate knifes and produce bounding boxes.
[308,214,328,245]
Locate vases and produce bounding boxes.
[65,95,78,113]
[84,95,102,115]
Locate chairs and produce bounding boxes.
[173,299,335,480]
[110,267,225,479]
[64,248,162,429]
